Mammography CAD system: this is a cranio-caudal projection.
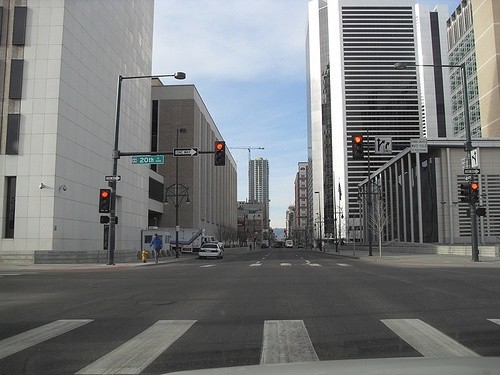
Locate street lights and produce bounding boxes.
[164,129,191,258]
[358,127,381,256]
[107,72,186,265]
[394,62,479,262]
[314,192,327,251]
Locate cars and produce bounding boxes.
[199,243,224,259]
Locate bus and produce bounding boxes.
[285,240,293,248]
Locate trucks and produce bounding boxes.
[149,226,224,253]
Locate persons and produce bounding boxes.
[149,233,163,264]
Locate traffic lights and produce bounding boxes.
[100,216,110,223]
[352,134,364,160]
[476,208,486,216]
[470,182,479,203]
[460,182,469,203]
[215,141,225,166]
[99,189,111,213]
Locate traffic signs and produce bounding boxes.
[465,175,472,181]
[173,147,198,157]
[105,175,121,181]
[464,168,480,174]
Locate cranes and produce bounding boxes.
[229,147,265,160]
